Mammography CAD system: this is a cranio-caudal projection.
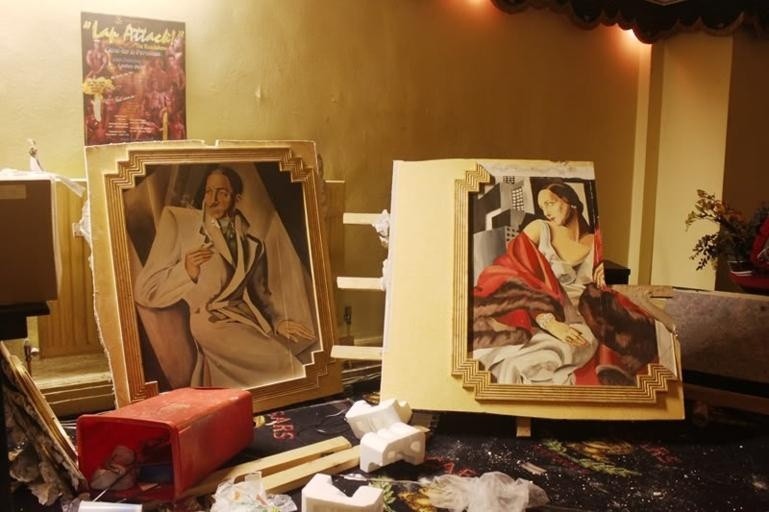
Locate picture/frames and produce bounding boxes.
[101,146,346,418]
[449,164,680,403]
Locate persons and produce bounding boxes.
[84,34,110,78]
[130,168,317,379]
[136,51,187,138]
[480,182,614,386]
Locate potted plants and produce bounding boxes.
[683,189,767,291]
